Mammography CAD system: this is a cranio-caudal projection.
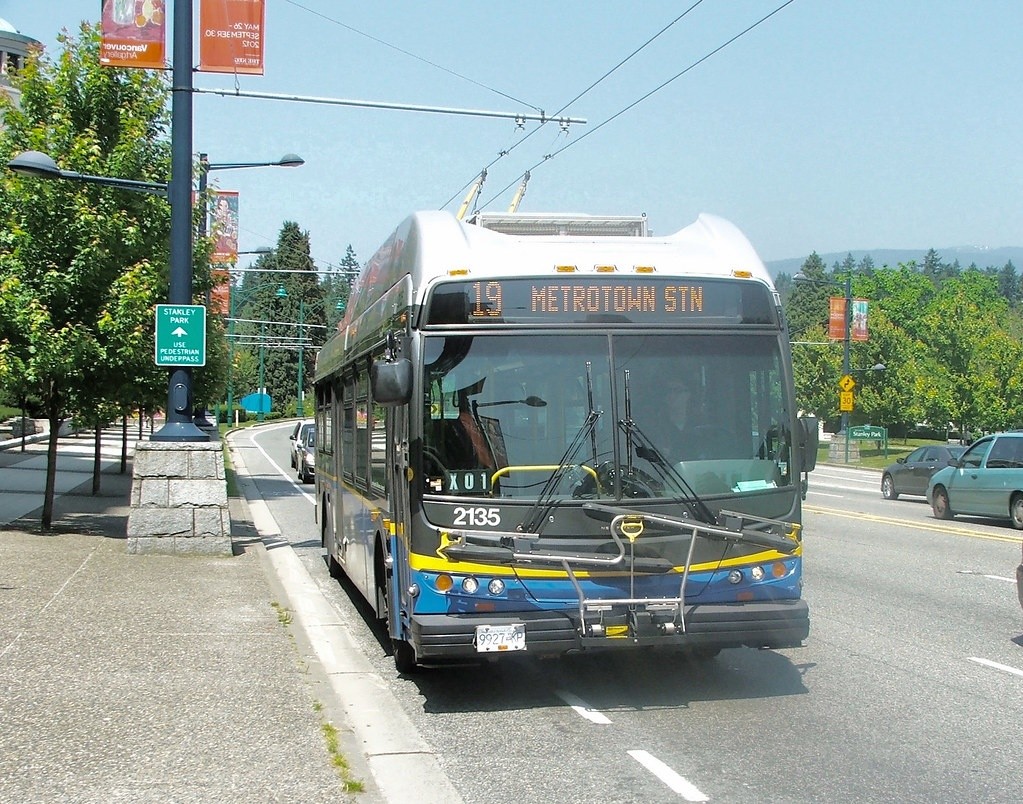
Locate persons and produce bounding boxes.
[658,377,738,459]
[967,430,973,446]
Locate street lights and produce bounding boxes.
[296,297,345,417]
[6,150,212,443]
[227,283,288,428]
[237,247,271,254]
[473,397,548,494]
[193,154,305,427]
[792,274,887,434]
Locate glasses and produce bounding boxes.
[665,387,692,396]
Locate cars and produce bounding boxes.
[290,420,331,471]
[297,428,331,484]
[926,433,1023,530]
[881,446,986,500]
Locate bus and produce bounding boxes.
[311,210,817,674]
